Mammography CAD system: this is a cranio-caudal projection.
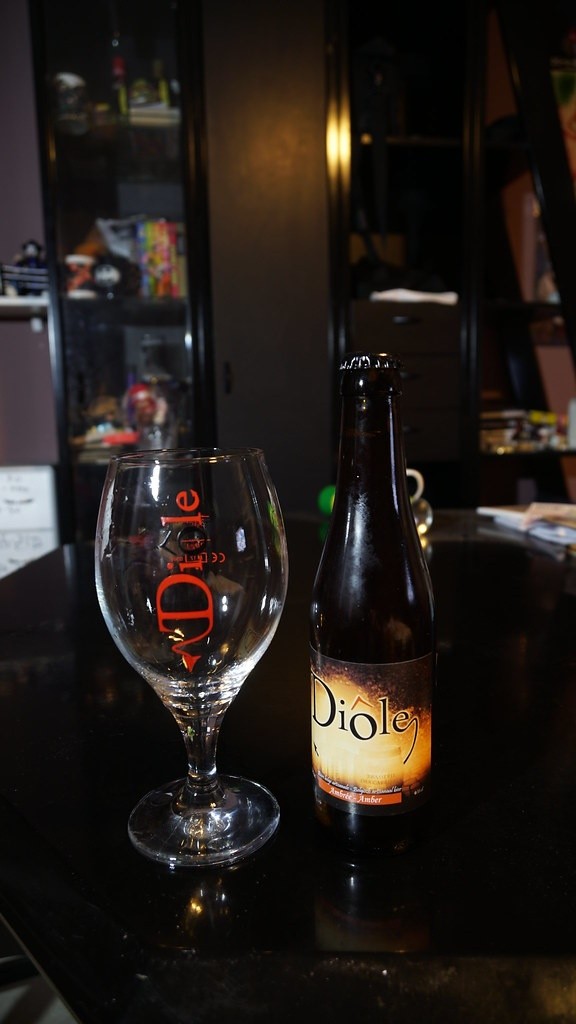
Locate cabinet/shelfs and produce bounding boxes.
[344,289,469,465]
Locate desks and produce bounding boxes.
[0,596,575,1024]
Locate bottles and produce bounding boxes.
[309,352,440,819]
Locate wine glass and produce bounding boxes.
[91,445,288,869]
